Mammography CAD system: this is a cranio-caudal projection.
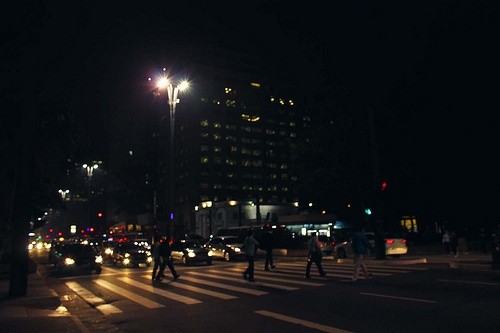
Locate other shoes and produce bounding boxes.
[174,274,181,279]
[156,279,161,281]
[306,276,311,279]
[248,279,256,282]
[161,274,165,277]
[243,272,247,280]
[322,271,327,277]
[271,265,275,268]
[265,269,270,272]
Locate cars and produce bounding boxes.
[48,238,103,275]
[100,240,152,268]
[316,235,335,254]
[168,239,211,267]
[335,231,408,259]
[206,236,248,262]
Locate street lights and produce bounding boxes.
[158,77,190,236]
[83,162,100,192]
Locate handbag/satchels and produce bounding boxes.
[311,249,322,260]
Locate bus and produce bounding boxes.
[218,222,291,250]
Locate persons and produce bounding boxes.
[441,230,458,255]
[305,232,326,279]
[260,228,276,271]
[151,233,180,281]
[479,232,488,255]
[352,226,370,281]
[242,229,258,282]
